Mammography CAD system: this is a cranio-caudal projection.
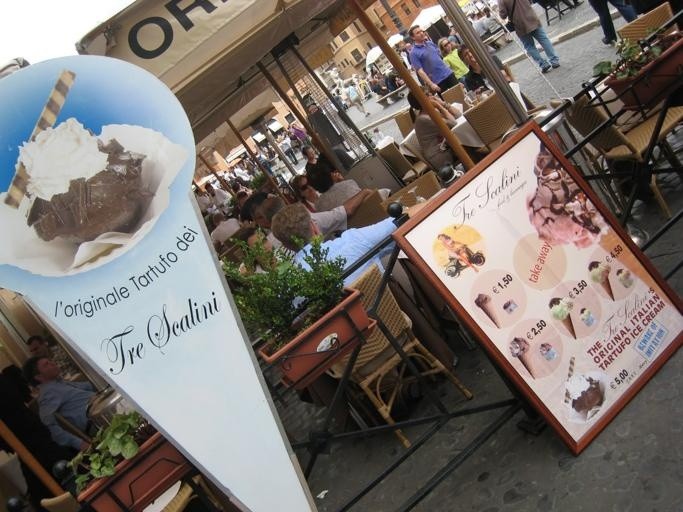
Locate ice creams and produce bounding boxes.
[549,297,578,340]
[0,55,319,512]
[474,293,503,327]
[510,337,537,379]
[589,261,616,302]
[527,141,673,307]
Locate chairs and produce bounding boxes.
[0,1,682,512]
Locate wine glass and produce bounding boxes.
[406,186,426,203]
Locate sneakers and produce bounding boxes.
[503,38,514,45]
[365,112,371,117]
[575,0,585,6]
[549,56,561,70]
[399,92,406,100]
[291,159,299,166]
[600,34,619,47]
[539,62,553,74]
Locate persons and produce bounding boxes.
[193,0,683,413]
[0,336,100,512]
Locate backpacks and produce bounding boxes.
[386,80,396,91]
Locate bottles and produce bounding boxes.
[462,86,493,107]
[363,128,384,150]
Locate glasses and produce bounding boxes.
[442,41,452,48]
[297,180,313,192]
[424,89,433,97]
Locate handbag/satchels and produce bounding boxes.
[505,21,516,32]
